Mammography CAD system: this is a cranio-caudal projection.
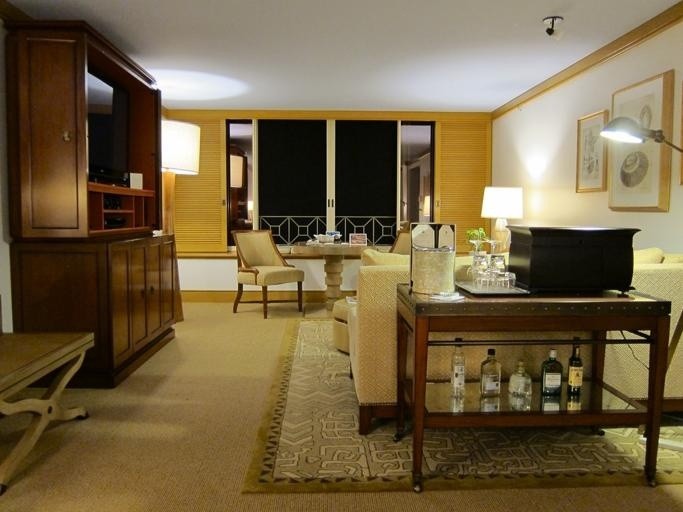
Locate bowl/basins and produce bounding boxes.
[313,235,342,243]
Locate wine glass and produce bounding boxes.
[470,237,501,255]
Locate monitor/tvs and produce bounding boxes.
[87,63,130,187]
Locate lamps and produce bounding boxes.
[230,155,243,223]
[423,196,430,216]
[161,119,200,234]
[600,117,683,154]
[542,16,565,41]
[480,186,523,253]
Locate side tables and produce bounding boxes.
[304,247,376,319]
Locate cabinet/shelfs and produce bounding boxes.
[7,18,163,243]
[9,235,175,388]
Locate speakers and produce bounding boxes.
[129,173,143,190]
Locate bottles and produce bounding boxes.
[566,400,582,414]
[480,398,503,413]
[449,396,466,413]
[541,398,560,413]
[508,397,534,412]
[450,337,465,395]
[568,346,584,403]
[334,235,341,244]
[507,360,534,396]
[480,348,501,397]
[540,348,563,398]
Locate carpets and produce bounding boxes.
[240,319,683,494]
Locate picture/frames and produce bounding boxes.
[609,69,674,212]
[576,109,609,193]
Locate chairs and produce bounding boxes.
[389,231,411,255]
[0,294,95,495]
[231,230,304,319]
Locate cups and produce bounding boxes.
[472,257,516,292]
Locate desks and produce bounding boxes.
[395,282,672,494]
